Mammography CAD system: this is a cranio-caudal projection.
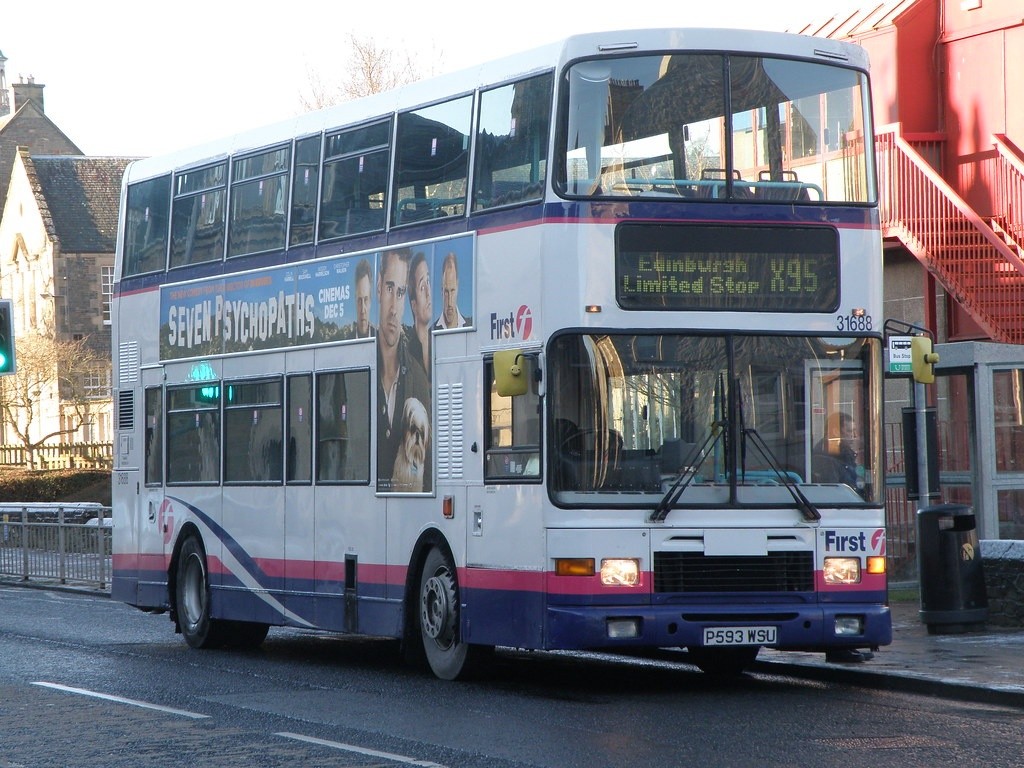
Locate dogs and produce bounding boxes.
[390,396,432,492]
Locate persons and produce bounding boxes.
[811,411,870,505]
[325,246,472,492]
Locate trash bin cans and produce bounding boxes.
[915,503,990,637]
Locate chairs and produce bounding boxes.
[651,179,696,198]
[697,168,752,199]
[397,198,448,226]
[755,171,810,202]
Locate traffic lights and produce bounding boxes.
[0,299,16,376]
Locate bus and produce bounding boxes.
[110,28,895,681]
[0,502,103,522]
